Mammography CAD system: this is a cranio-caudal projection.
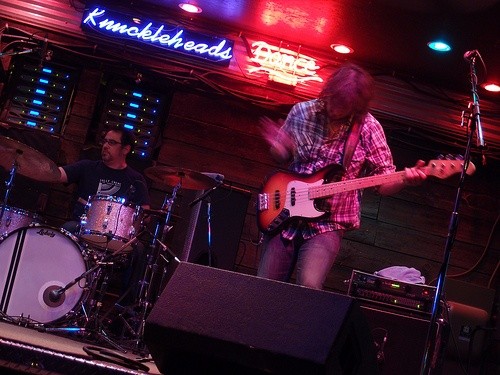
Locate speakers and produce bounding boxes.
[114,185,252,339]
[143,260,450,375]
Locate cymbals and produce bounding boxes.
[143,207,184,220]
[143,166,216,190]
[0,135,61,181]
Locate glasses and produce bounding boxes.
[100,139,122,146]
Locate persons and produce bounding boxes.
[58,125,165,338]
[255,64,428,291]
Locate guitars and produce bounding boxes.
[256,154,476,236]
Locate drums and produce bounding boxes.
[0,203,46,241]
[0,223,93,329]
[76,193,143,254]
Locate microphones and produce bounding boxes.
[188,187,217,207]
[463,50,478,62]
[49,289,62,304]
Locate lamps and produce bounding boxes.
[178,0,203,13]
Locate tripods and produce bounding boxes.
[38,181,184,355]
